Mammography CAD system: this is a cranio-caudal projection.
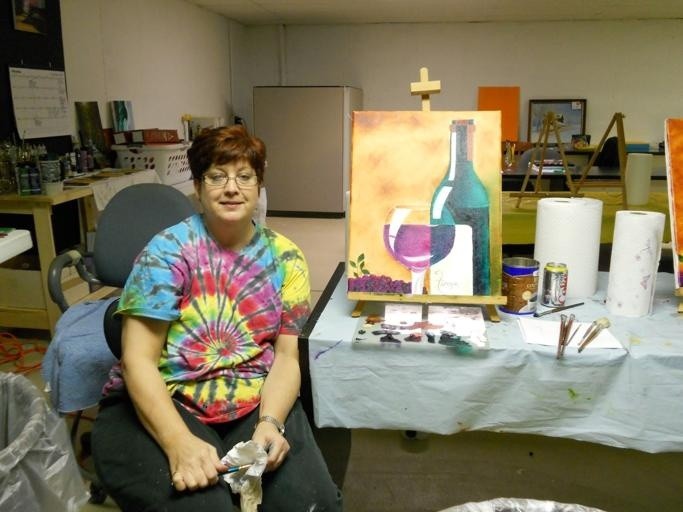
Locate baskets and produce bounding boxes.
[109,142,194,187]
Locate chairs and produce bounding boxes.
[46,183,199,505]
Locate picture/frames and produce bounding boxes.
[528,98,586,147]
[110,100,134,133]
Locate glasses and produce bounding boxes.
[201,170,260,189]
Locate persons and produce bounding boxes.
[92,125,344,512]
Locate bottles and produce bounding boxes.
[429,120,492,296]
[20,146,95,196]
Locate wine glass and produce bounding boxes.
[382,203,456,295]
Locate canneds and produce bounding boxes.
[542,262,568,308]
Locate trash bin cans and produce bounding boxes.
[440,497,607,512]
[0,371,90,512]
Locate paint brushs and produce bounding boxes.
[171,464,253,486]
[557,314,610,359]
[534,301,586,317]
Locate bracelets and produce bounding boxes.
[252,416,286,435]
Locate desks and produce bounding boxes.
[499,144,676,244]
[296,263,683,454]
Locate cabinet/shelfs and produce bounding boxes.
[0,166,157,344]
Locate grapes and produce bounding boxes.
[348,274,412,293]
